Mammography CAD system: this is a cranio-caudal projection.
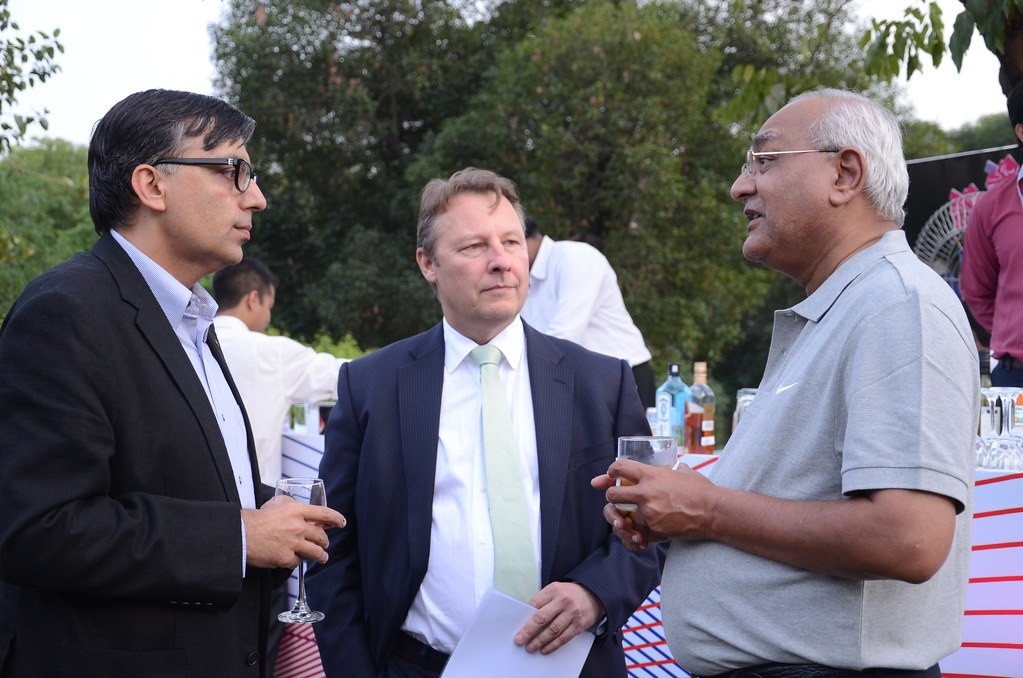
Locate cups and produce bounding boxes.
[616,436,677,511]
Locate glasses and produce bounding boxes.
[150,155,259,194]
[741,150,839,175]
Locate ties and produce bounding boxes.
[467,346,542,611]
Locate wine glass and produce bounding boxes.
[273,477,327,625]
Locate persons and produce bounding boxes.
[1,87,346,677]
[588,89,978,678]
[960,80,1023,387]
[520,215,656,414]
[303,166,674,678]
[212,256,353,505]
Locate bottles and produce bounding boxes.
[683,362,716,455]
[654,363,692,455]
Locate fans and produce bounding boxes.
[910,191,1007,373]
